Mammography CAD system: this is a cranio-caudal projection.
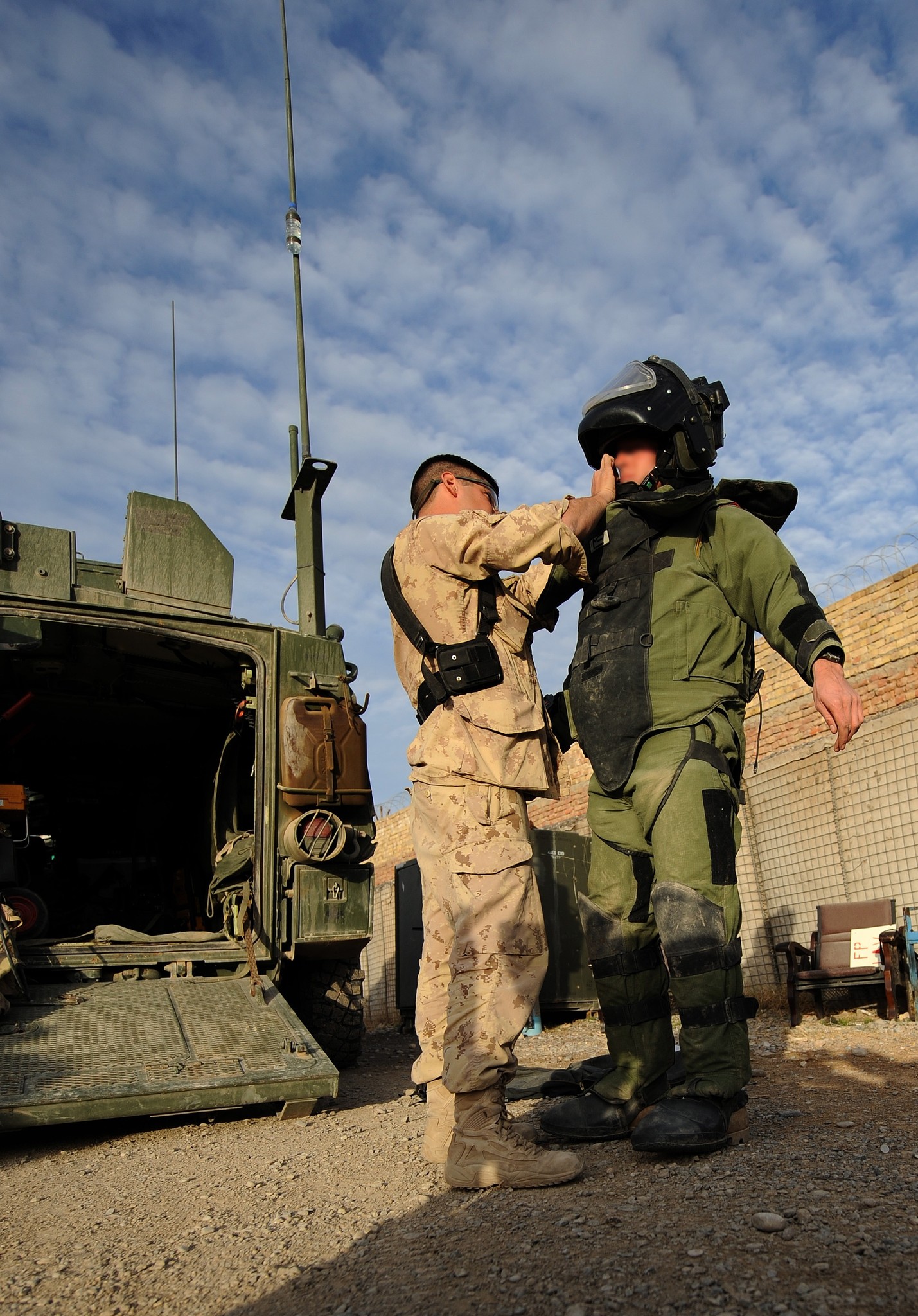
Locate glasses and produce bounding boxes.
[455,476,499,511]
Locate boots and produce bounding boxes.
[444,1080,585,1189]
[421,1078,538,1164]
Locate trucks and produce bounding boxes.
[0,0,379,1130]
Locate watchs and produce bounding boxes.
[818,650,842,665]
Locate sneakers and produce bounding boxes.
[631,1090,751,1155]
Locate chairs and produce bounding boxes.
[775,898,906,1028]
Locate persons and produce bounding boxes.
[541,358,867,1150]
[378,454,616,1189]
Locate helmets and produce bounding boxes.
[578,355,731,490]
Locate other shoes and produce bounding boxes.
[540,1072,671,1139]
